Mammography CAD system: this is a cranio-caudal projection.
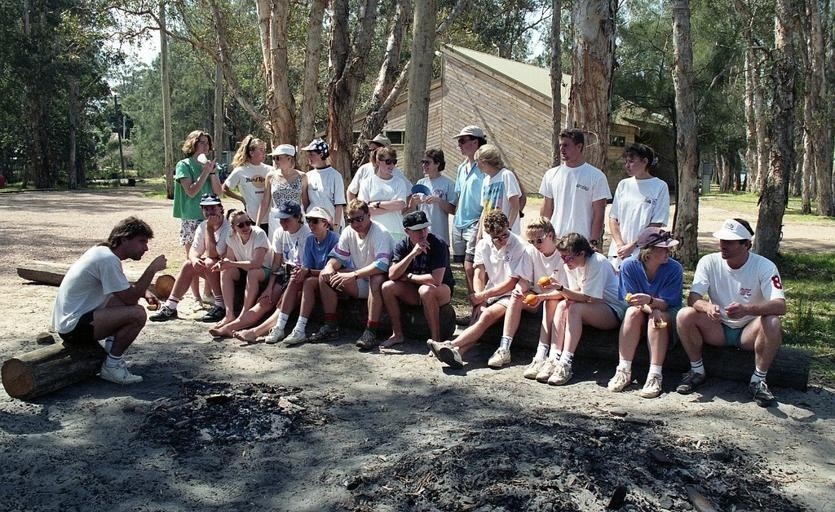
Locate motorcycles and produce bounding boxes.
[215,162,228,182]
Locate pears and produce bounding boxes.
[526,294,537,305]
[540,277,550,286]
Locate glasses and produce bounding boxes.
[526,231,549,245]
[232,219,251,228]
[489,232,510,242]
[201,193,217,199]
[379,157,398,165]
[306,217,325,224]
[344,213,366,224]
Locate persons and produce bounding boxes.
[51,215,168,386]
[675,217,786,404]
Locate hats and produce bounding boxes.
[712,218,753,245]
[366,133,392,149]
[199,192,222,208]
[273,200,301,219]
[635,226,680,250]
[303,206,331,225]
[301,138,329,155]
[451,123,487,139]
[267,144,296,157]
[401,210,432,232]
[411,184,431,203]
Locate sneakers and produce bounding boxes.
[675,369,709,394]
[355,325,377,350]
[534,359,560,382]
[265,326,285,344]
[202,305,226,322]
[307,322,341,344]
[98,358,144,385]
[438,344,464,370]
[746,378,775,408]
[282,329,307,345]
[148,305,178,322]
[192,292,216,312]
[522,355,549,380]
[640,371,664,397]
[606,366,633,393]
[545,363,574,387]
[425,336,452,362]
[487,347,512,368]
